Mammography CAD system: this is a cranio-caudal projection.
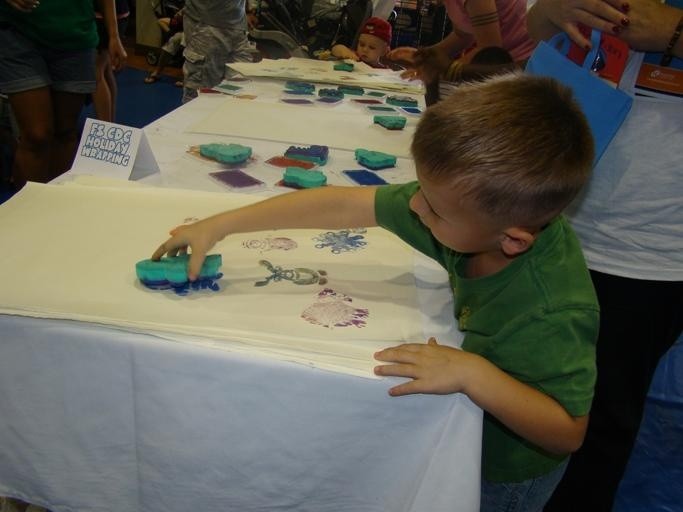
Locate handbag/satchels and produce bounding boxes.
[524,29,633,171]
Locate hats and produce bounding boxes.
[360,17,392,45]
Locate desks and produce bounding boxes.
[1,56,484,512]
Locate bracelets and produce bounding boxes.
[443,60,464,81]
[467,12,500,27]
[660,18,683,68]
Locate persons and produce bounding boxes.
[150,70,601,511]
[181,0,252,103]
[143,0,185,87]
[92,1,131,122]
[384,0,537,82]
[331,17,393,69]
[525,1,682,510]
[460,46,513,84]
[0,0,128,193]
[308,0,348,29]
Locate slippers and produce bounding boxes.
[144,75,160,84]
